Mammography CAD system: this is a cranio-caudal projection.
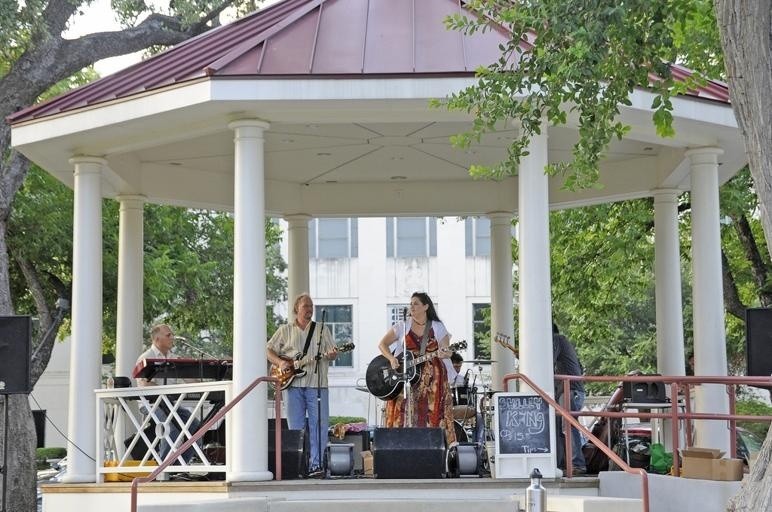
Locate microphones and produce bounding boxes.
[174,336,185,340]
[463,370,469,378]
[404,307,408,321]
[321,308,326,322]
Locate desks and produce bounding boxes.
[598,398,689,472]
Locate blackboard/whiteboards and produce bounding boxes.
[493,392,556,458]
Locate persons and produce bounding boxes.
[135,324,204,481]
[378,291,459,446]
[513,322,587,474]
[450,351,485,459]
[267,292,341,479]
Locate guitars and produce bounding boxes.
[365,341,467,401]
[269,342,355,391]
[493,332,517,355]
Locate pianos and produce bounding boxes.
[132,358,232,379]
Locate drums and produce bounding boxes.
[450,384,477,418]
[452,419,469,445]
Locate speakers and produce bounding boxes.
[623,374,666,403]
[269,430,306,479]
[372,427,445,479]
[0,314,31,394]
[746,307,772,390]
[124,424,160,461]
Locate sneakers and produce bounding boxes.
[308,470,326,478]
[563,465,587,477]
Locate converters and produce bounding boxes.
[668,397,683,403]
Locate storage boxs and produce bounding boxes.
[681,444,745,480]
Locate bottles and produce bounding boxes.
[526,467,548,512]
[106,370,114,390]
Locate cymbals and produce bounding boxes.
[460,360,494,363]
[477,390,504,395]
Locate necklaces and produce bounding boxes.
[411,316,425,327]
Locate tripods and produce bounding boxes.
[486,394,495,441]
